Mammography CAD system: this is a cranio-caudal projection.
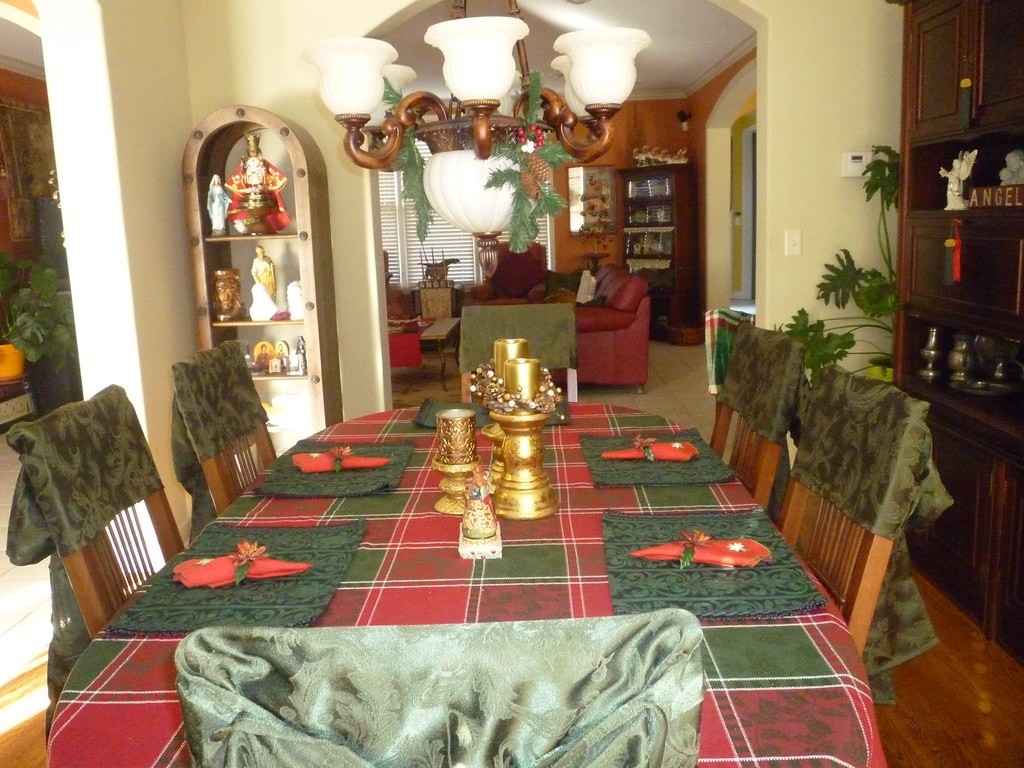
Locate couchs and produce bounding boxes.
[550,263,652,394]
[471,239,551,306]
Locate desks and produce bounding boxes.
[46,399,890,767]
[583,252,610,264]
[411,282,465,346]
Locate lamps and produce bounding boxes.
[303,0,651,280]
[676,110,692,132]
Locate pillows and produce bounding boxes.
[576,270,597,304]
[544,287,577,305]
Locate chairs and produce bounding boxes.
[172,608,704,768]
[172,340,278,544]
[711,320,955,707]
[5,385,187,744]
[459,303,579,403]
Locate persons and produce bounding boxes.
[223,130,291,232]
[939,149,979,211]
[251,246,276,304]
[206,175,235,230]
[296,336,307,369]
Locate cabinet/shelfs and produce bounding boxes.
[884,0,1024,667]
[615,164,698,343]
[181,104,343,434]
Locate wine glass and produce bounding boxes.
[919,326,940,375]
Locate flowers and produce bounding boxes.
[229,540,270,587]
[630,435,657,462]
[673,527,712,571]
[327,446,355,473]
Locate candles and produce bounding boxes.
[502,359,541,404]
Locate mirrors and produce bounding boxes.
[566,164,618,237]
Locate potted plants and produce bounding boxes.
[418,258,460,279]
[494,336,529,379]
[773,141,900,383]
[0,253,78,378]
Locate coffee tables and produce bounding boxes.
[388,317,461,392]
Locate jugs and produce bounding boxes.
[947,333,974,380]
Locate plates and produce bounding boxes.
[950,378,1013,394]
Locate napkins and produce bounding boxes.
[173,556,311,588]
[629,538,773,568]
[601,441,700,462]
[292,453,390,473]
